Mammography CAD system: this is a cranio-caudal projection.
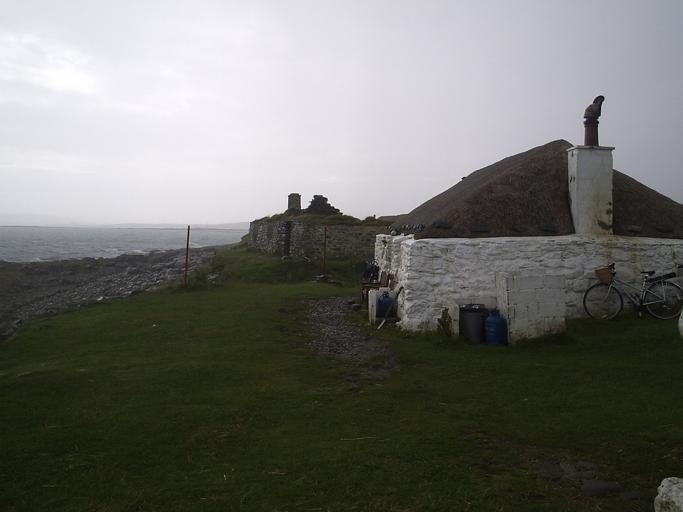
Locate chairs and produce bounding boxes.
[360,270,389,307]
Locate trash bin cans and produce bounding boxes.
[459,303,488,344]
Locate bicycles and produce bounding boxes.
[582,262,682,321]
